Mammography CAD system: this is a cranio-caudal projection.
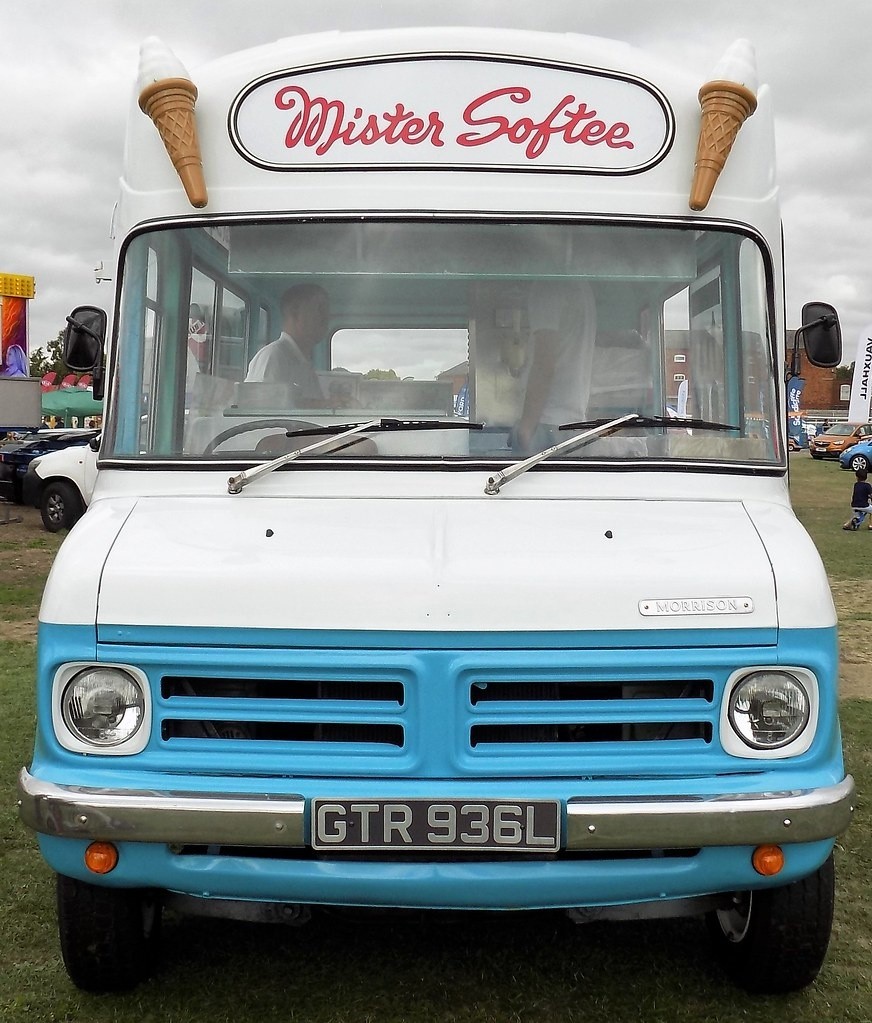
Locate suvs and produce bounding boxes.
[0,409,190,533]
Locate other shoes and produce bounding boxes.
[867,524,872,529]
[843,523,851,529]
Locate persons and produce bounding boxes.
[822,419,831,432]
[244,284,364,409]
[487,278,597,459]
[42,415,95,429]
[842,468,872,531]
[4,344,27,377]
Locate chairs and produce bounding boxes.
[309,370,462,420]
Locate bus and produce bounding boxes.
[22,27,855,995]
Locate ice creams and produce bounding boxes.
[689,37,759,208]
[136,36,208,210]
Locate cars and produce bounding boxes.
[810,422,872,472]
[789,438,802,452]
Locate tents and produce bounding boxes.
[42,386,104,429]
